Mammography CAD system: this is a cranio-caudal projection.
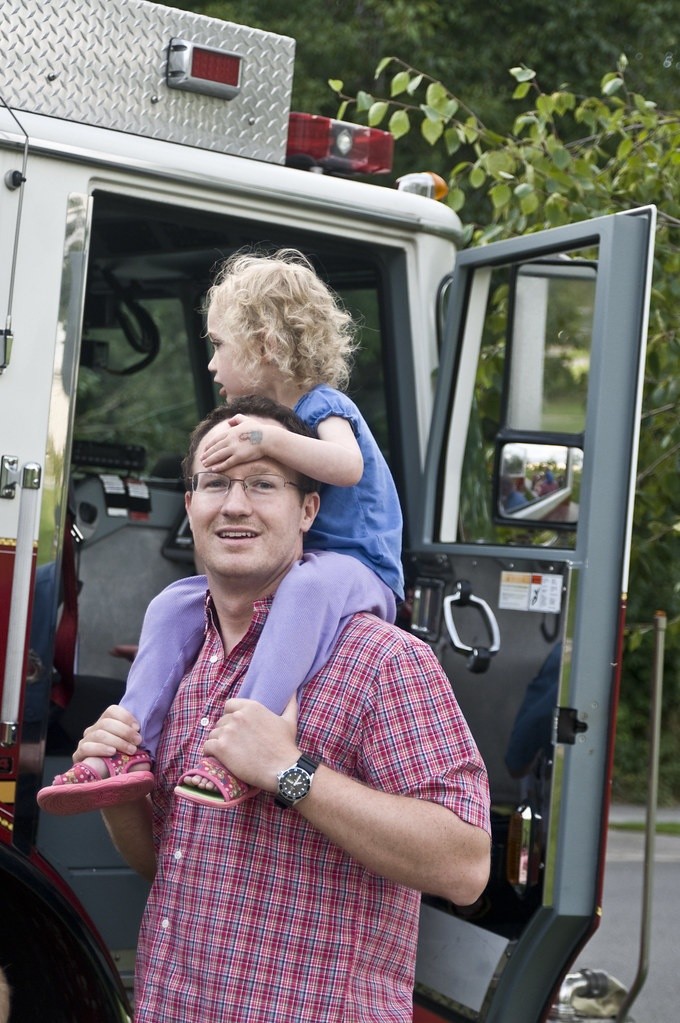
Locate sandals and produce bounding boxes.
[36,748,156,815]
[174,758,261,808]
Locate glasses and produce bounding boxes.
[188,471,307,502]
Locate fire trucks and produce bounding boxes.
[0,0,666,1023]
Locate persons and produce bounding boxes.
[500,456,564,512]
[72,396,492,1023]
[15,563,59,784]
[36,247,403,816]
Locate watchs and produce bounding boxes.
[275,754,320,809]
